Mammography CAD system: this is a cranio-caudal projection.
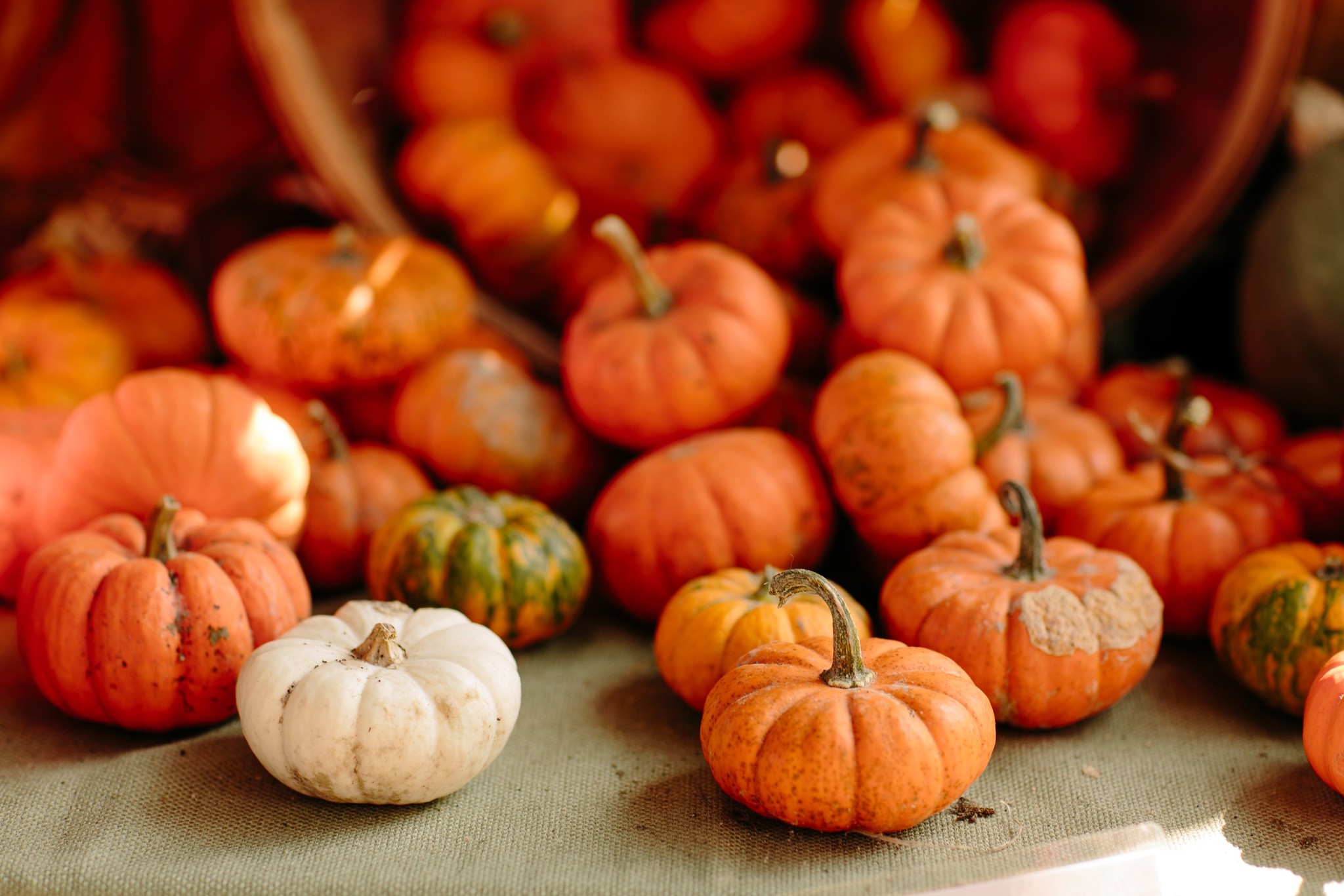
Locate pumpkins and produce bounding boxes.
[1301,651,1343,798]
[365,483,594,651]
[880,478,1161,729]
[15,494,313,732]
[3,2,1343,625]
[700,567,997,835]
[235,597,521,805]
[653,567,869,718]
[1053,356,1307,641]
[1206,539,1342,710]
